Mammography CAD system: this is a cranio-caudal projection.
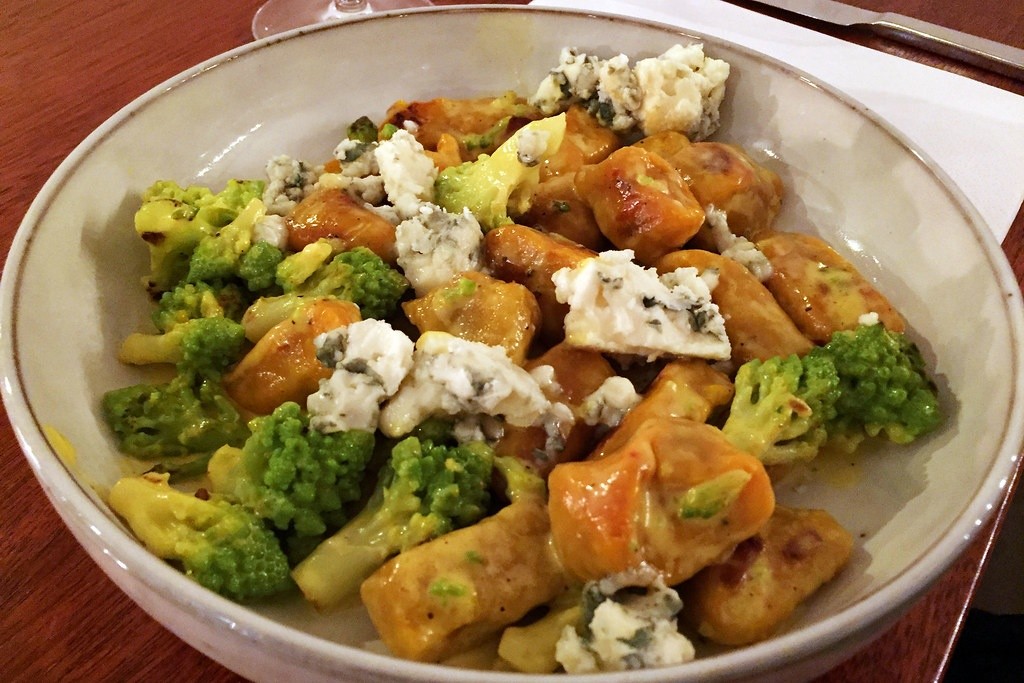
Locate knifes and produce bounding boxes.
[752,0,1024,79]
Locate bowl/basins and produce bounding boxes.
[0,4,1024,683]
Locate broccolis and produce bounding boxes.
[97,109,951,676]
